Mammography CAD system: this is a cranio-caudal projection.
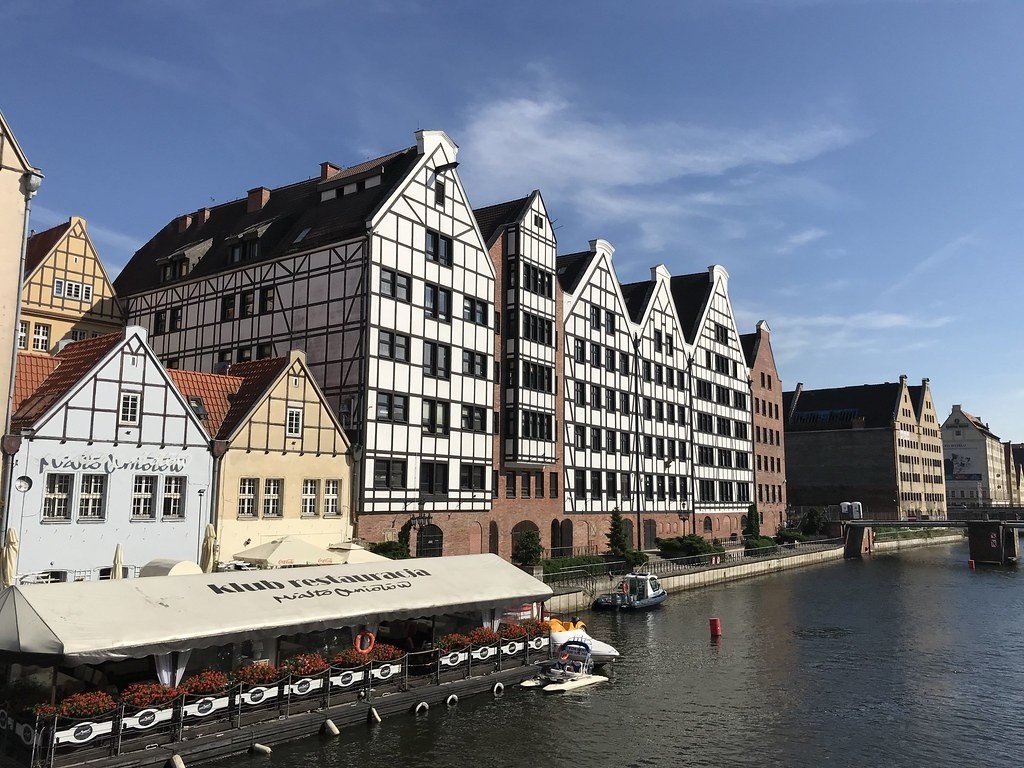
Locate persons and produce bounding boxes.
[623,581,629,594]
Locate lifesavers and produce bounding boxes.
[492,681,505,693]
[444,694,458,703]
[354,629,375,655]
[623,583,630,593]
[411,700,429,713]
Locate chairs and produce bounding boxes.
[434,614,459,631]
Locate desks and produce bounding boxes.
[21,671,80,698]
[280,640,305,657]
[410,618,445,635]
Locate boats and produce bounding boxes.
[593,572,669,610]
[548,618,620,665]
[520,635,609,692]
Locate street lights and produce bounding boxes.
[892,492,902,519]
[677,497,690,540]
[784,500,795,530]
[410,502,433,557]
[197,488,206,565]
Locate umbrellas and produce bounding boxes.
[233,536,343,564]
[0,528,19,588]
[199,523,217,573]
[327,543,392,564]
[110,544,122,579]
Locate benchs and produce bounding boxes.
[73,665,104,690]
